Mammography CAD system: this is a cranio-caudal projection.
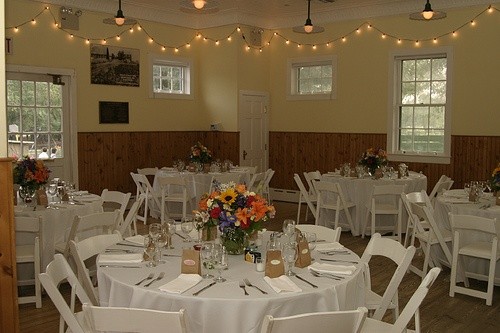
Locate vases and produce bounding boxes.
[27,184,45,197]
[220,218,249,256]
[197,162,212,171]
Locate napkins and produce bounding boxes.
[323,174,340,177]
[98,254,144,264]
[262,274,303,294]
[158,273,204,294]
[127,233,154,248]
[308,240,345,251]
[75,191,89,194]
[150,166,255,221]
[190,228,202,239]
[308,262,358,276]
[445,198,471,204]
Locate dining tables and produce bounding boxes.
[92,224,373,333]
[320,169,427,235]
[435,182,500,288]
[16,193,103,287]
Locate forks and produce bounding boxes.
[238,279,268,295]
[135,272,165,287]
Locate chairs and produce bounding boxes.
[15,166,500,333]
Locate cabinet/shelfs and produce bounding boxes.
[0,156,20,333]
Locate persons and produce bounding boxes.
[51,148,57,158]
[39,148,49,158]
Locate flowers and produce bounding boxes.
[14,158,50,184]
[188,140,214,162]
[192,180,276,230]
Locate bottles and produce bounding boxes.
[243,244,261,263]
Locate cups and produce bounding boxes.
[256,257,265,271]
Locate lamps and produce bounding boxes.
[102,0,137,27]
[292,0,325,35]
[179,0,222,15]
[408,0,448,22]
[56,5,84,32]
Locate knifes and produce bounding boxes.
[100,264,141,268]
[320,258,359,264]
[193,282,217,296]
[292,272,318,288]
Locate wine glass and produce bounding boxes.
[282,242,298,276]
[198,163,203,174]
[381,165,395,179]
[45,177,75,210]
[267,219,306,263]
[181,217,194,242]
[464,179,493,206]
[304,232,317,262]
[144,219,176,268]
[172,158,185,173]
